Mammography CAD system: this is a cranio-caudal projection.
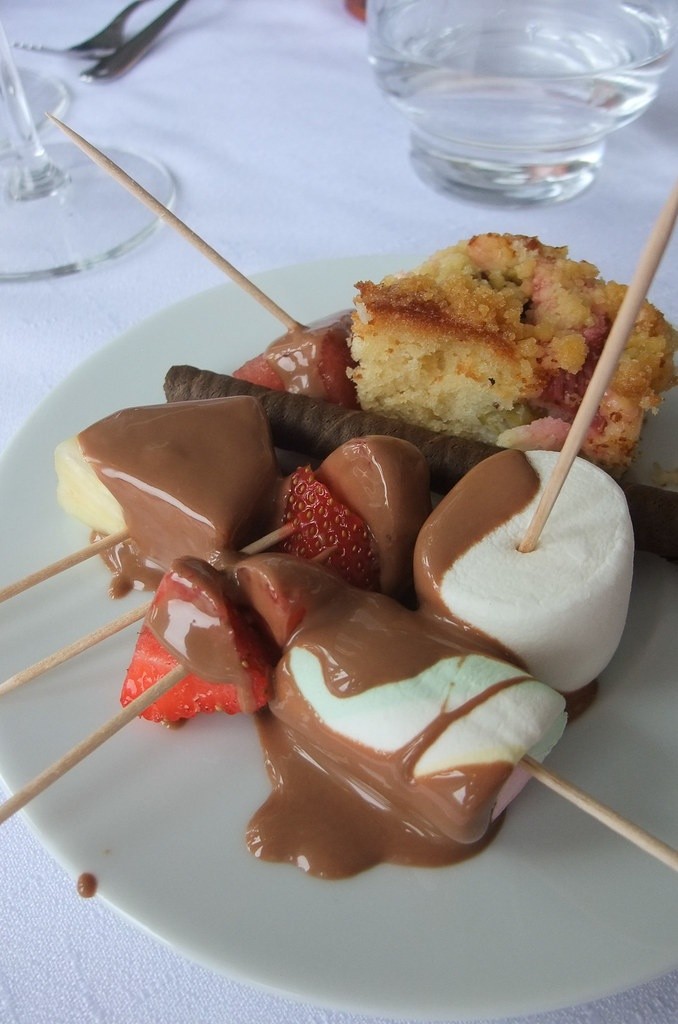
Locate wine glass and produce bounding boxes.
[0,25,176,280]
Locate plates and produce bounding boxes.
[0,253,677,1024]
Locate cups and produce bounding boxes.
[364,0,678,207]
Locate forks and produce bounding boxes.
[12,1,152,59]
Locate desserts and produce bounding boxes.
[48,233,678,850]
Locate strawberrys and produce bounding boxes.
[118,555,273,727]
[267,462,381,592]
